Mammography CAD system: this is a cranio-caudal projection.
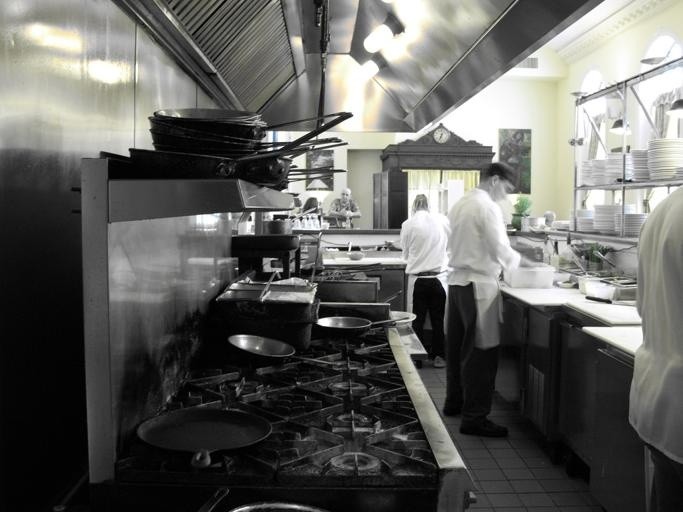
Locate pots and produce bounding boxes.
[138,406,272,468]
[315,316,409,337]
[98,108,352,189]
[228,334,345,367]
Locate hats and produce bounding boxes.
[486,161,518,187]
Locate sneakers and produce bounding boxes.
[443,398,508,437]
[433,356,446,367]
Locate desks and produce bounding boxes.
[318,214,360,229]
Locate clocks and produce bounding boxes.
[433,127,450,143]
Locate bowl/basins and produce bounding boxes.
[347,251,364,260]
[390,311,417,330]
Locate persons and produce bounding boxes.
[330,186,361,228]
[627,184,683,512]
[443,162,546,436]
[400,195,447,369]
[303,198,319,214]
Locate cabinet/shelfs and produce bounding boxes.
[567,55,683,243]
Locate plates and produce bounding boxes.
[552,138,683,237]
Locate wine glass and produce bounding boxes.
[293,213,320,230]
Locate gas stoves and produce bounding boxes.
[132,323,442,482]
